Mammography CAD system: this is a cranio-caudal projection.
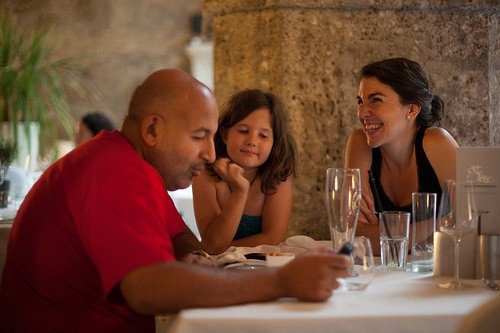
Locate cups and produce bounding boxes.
[480,235,500,290]
[340,237,375,291]
[266,252,296,267]
[325,167,361,277]
[412,192,437,272]
[379,211,411,271]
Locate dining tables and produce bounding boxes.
[169,227,500,333]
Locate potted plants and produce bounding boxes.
[0,18,113,210]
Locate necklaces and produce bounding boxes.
[229,173,258,193]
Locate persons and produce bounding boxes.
[340,57,460,257]
[0,69,355,333]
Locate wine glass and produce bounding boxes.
[437,180,478,290]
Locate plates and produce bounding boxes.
[223,262,266,268]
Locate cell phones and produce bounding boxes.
[244,253,266,260]
